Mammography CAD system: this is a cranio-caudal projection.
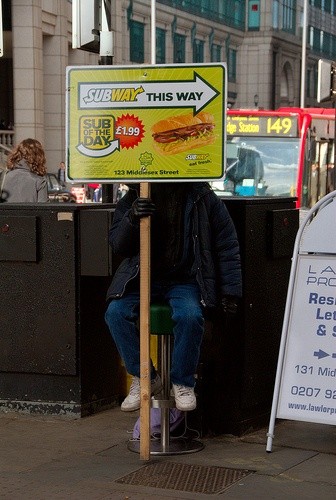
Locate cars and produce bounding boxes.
[41,173,65,194]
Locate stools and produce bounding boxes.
[128,305,205,454]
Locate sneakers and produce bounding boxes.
[120,375,161,411]
[169,384,197,411]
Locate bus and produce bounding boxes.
[211,102,336,226]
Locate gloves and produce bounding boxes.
[219,297,241,312]
[130,197,157,218]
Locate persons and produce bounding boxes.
[0,137,51,203]
[105,182,244,410]
[57,161,68,190]
